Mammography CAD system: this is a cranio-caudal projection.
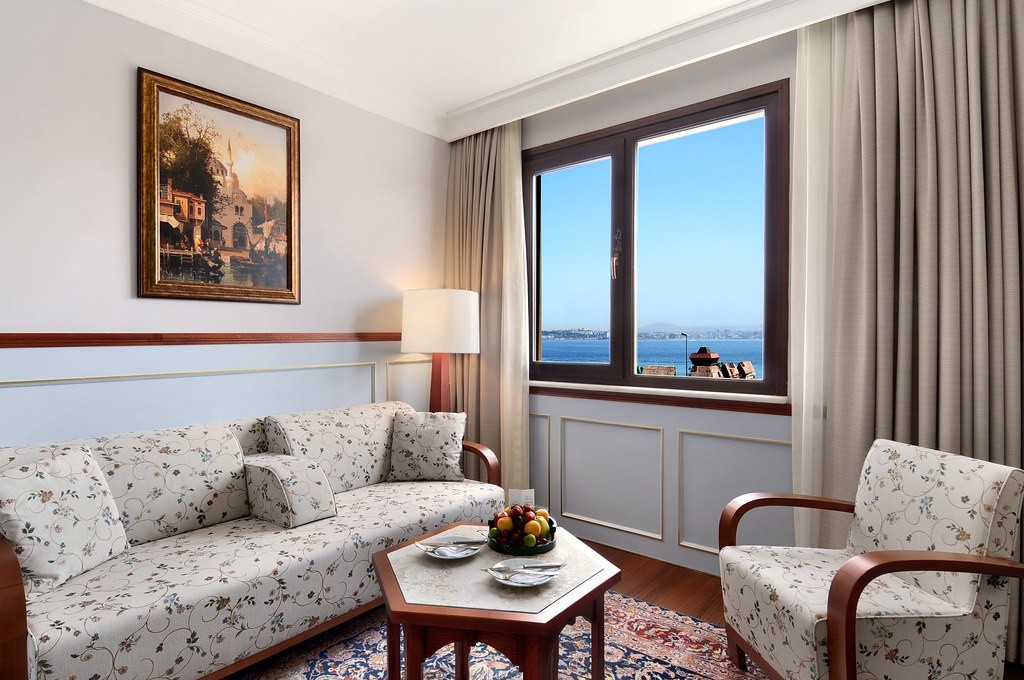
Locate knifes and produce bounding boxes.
[478,562,565,569]
[420,541,488,545]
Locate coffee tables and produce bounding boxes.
[370,520,621,680]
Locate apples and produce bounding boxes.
[523,534,536,547]
[488,527,498,538]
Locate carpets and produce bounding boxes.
[236,586,772,679]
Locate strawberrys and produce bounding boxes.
[508,504,535,525]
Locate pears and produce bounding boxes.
[534,515,549,536]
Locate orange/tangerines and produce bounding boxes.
[524,520,541,537]
[497,517,513,532]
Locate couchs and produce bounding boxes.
[715,437,1023,680]
[0,399,507,680]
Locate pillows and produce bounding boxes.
[0,444,133,594]
[386,409,467,482]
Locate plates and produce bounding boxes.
[426,536,481,559]
[492,558,558,587]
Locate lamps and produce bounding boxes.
[398,288,483,413]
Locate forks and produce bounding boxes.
[486,569,559,580]
[415,541,480,551]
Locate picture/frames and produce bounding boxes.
[137,66,303,307]
